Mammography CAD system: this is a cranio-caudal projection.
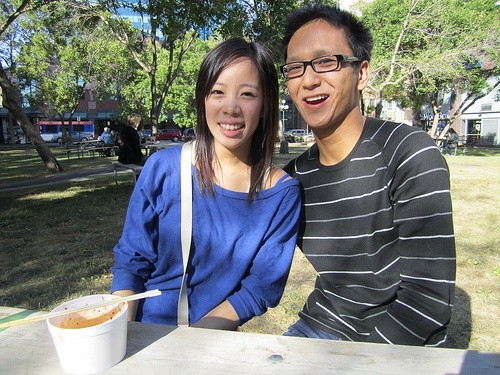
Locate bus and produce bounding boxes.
[34,120,95,143]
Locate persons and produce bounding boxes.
[445,128,458,154]
[118,113,149,182]
[101,127,120,156]
[111,37,302,330]
[280,7,457,347]
[141,130,146,143]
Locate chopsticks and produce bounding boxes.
[0,288,161,327]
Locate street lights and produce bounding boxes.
[279,100,289,131]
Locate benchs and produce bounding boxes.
[112,160,141,187]
[449,146,458,156]
[63,145,120,161]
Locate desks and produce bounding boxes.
[73,141,95,159]
[461,133,481,147]
[91,139,104,155]
[141,142,181,157]
[0,307,500,375]
[433,137,448,154]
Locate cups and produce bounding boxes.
[46,294,128,375]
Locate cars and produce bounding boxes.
[138,128,163,141]
[284,129,305,138]
[148,129,182,142]
[183,128,196,141]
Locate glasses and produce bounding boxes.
[280,54,363,80]
